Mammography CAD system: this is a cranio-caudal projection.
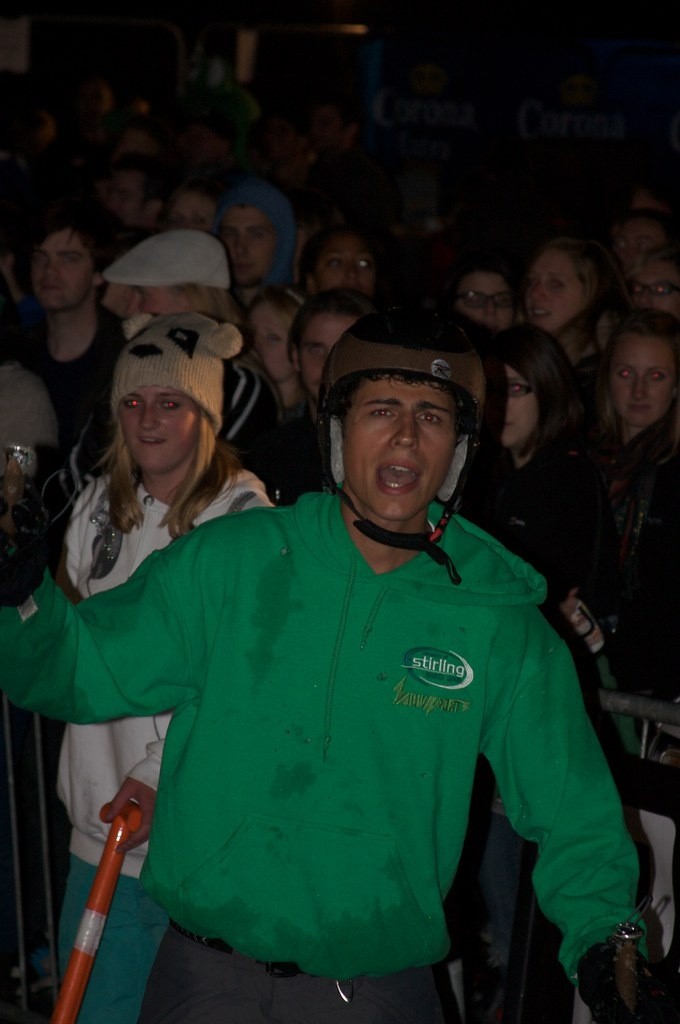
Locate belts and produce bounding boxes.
[168,917,303,978]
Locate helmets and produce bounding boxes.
[322,307,486,446]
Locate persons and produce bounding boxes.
[0,35,680,1024]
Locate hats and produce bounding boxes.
[211,176,296,284]
[111,311,243,438]
[102,228,231,288]
[0,360,58,447]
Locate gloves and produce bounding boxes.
[575,941,670,1024]
[0,470,49,608]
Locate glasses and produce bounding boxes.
[610,363,678,386]
[629,281,680,297]
[298,342,328,368]
[489,382,534,397]
[615,234,657,255]
[456,291,516,307]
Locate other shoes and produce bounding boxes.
[468,942,502,1018]
